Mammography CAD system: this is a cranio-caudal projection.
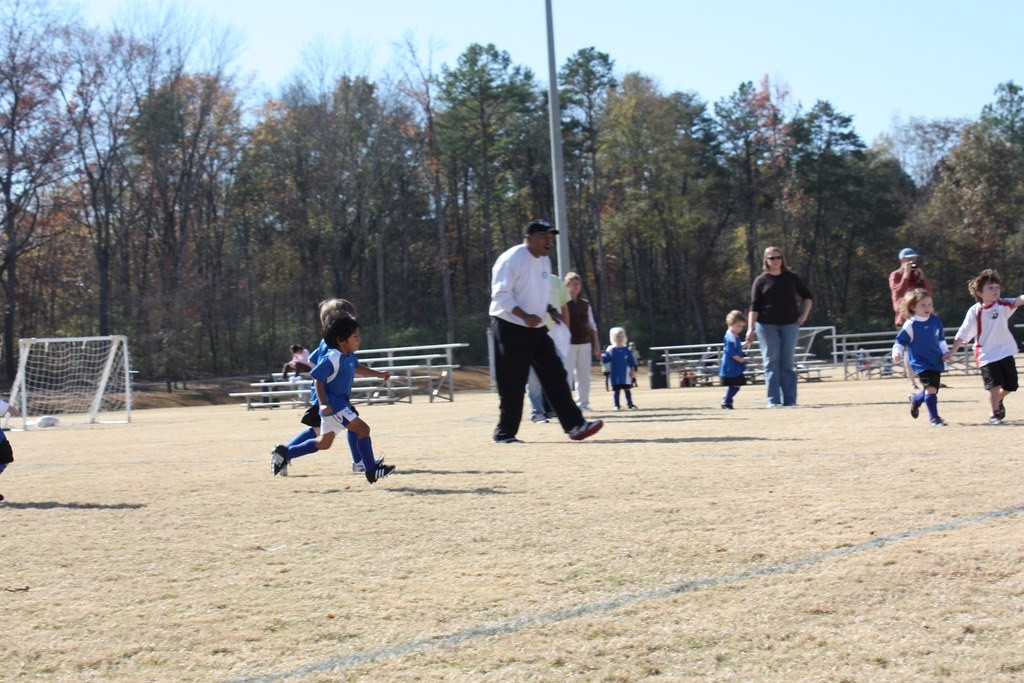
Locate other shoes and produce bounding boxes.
[550,412,559,423]
[629,405,636,409]
[533,414,548,424]
[614,405,621,409]
[722,398,733,411]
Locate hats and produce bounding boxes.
[899,249,920,261]
[524,220,558,236]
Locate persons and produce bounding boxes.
[700,345,718,385]
[940,269,1024,425]
[280,299,383,477]
[489,218,602,442]
[272,314,395,483]
[748,246,814,409]
[0,401,22,502]
[719,310,752,409]
[523,271,640,424]
[888,248,949,388]
[277,344,313,382]
[892,288,948,427]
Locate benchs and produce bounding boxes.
[228,343,469,409]
[649,327,1024,386]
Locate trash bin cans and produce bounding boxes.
[261,379,280,408]
[648,359,667,389]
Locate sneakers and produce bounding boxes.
[279,465,288,478]
[570,419,602,440]
[352,456,385,475]
[991,397,1006,426]
[365,463,396,482]
[273,446,289,474]
[496,436,522,445]
[909,393,920,418]
[932,417,946,427]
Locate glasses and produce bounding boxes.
[767,255,782,260]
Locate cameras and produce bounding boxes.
[910,262,920,269]
